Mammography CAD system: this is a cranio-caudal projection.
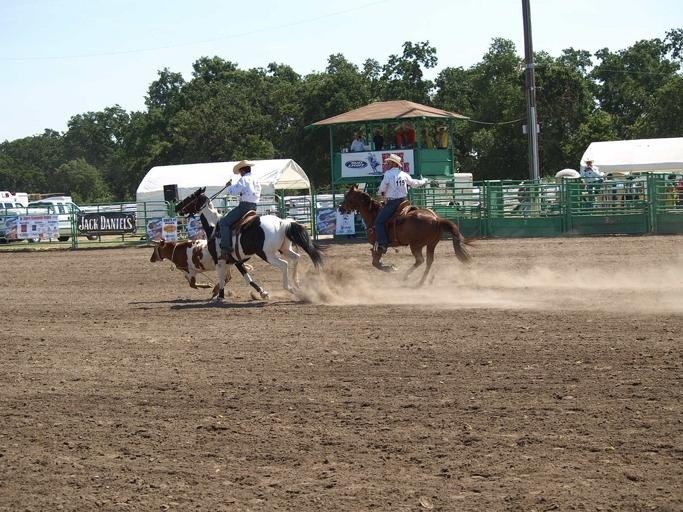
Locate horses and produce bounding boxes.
[338,184,479,291]
[173,186,330,304]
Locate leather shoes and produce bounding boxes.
[370,246,386,254]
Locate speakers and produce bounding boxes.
[163,184,179,204]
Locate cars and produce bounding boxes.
[473,185,487,213]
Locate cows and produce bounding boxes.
[148,237,256,289]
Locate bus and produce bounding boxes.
[223,194,345,223]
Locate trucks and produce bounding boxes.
[0,202,41,242]
[0,190,29,208]
[355,172,473,217]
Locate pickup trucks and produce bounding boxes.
[0,200,98,241]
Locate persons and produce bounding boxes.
[420,127,434,149]
[433,124,448,148]
[580,157,602,194]
[374,128,383,151]
[373,153,428,253]
[351,132,364,152]
[607,170,683,210]
[219,160,262,261]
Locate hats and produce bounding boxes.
[436,125,448,132]
[585,158,594,164]
[233,160,254,174]
[385,154,403,167]
